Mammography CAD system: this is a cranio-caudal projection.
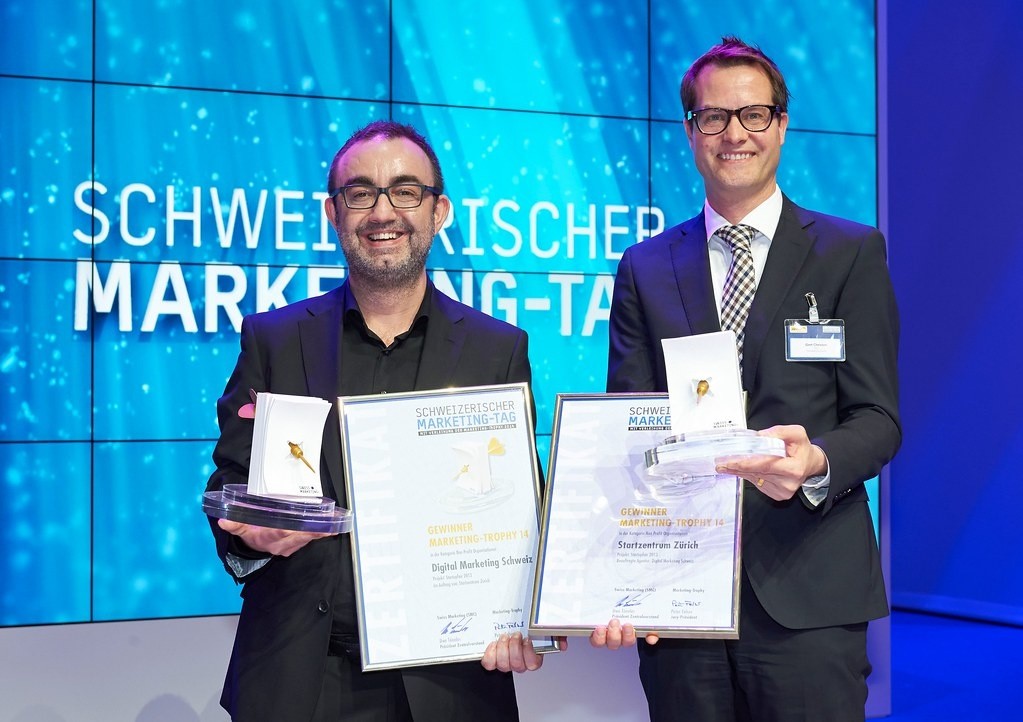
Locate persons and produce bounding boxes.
[589,41,903,722]
[203,122,569,722]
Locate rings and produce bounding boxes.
[756,478,764,487]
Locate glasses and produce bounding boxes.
[329,184,440,210]
[687,104,781,135]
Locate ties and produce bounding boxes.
[714,225,758,390]
